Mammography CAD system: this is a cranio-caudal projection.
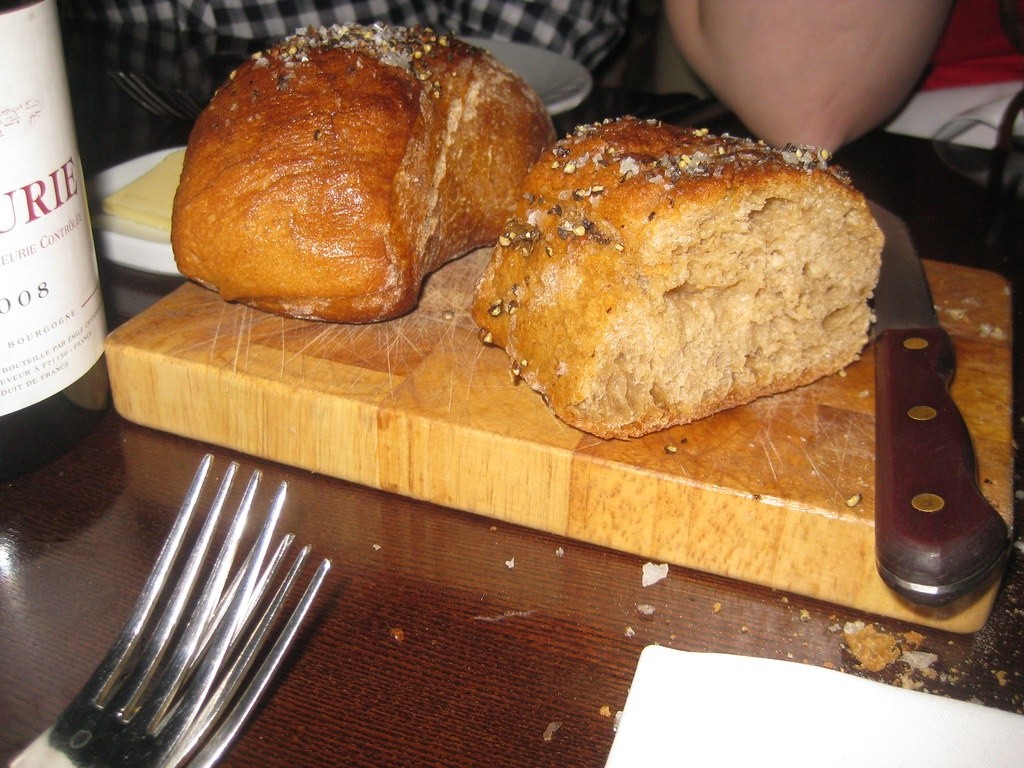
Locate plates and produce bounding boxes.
[455,36,593,115]
[85,146,190,276]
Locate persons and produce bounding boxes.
[76,0,1024,165]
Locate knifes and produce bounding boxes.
[866,199,1008,605]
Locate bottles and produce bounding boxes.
[1,0,113,481]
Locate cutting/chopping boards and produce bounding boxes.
[107,255,1016,634]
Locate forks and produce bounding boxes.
[111,70,202,127]
[153,534,332,767]
[5,454,290,767]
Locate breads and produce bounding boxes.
[473,115,883,440]
[169,22,559,326]
